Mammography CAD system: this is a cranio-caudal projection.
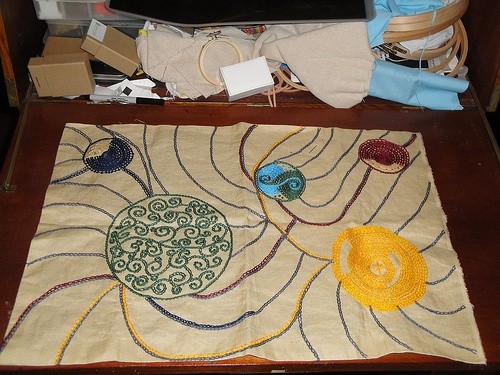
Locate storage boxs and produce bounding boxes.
[81,18,139,76]
[33,0,145,38]
[28,53,96,97]
[40,35,98,60]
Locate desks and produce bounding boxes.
[0,99,500,375]
